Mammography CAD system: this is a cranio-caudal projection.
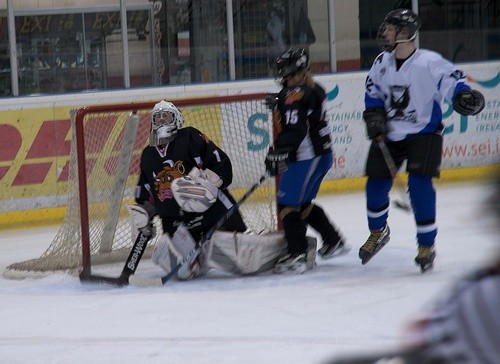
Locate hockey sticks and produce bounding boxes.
[376,134,413,213]
[79,231,151,289]
[157,171,271,287]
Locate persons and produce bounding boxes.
[359,10,485,273]
[265,47,345,273]
[128,100,256,281]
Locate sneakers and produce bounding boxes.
[274,253,307,275]
[359,224,390,265]
[414,242,435,273]
[317,236,345,259]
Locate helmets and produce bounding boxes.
[149,100,183,138]
[273,47,308,77]
[377,9,422,53]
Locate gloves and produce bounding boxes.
[265,146,288,175]
[452,90,485,117]
[363,105,388,139]
